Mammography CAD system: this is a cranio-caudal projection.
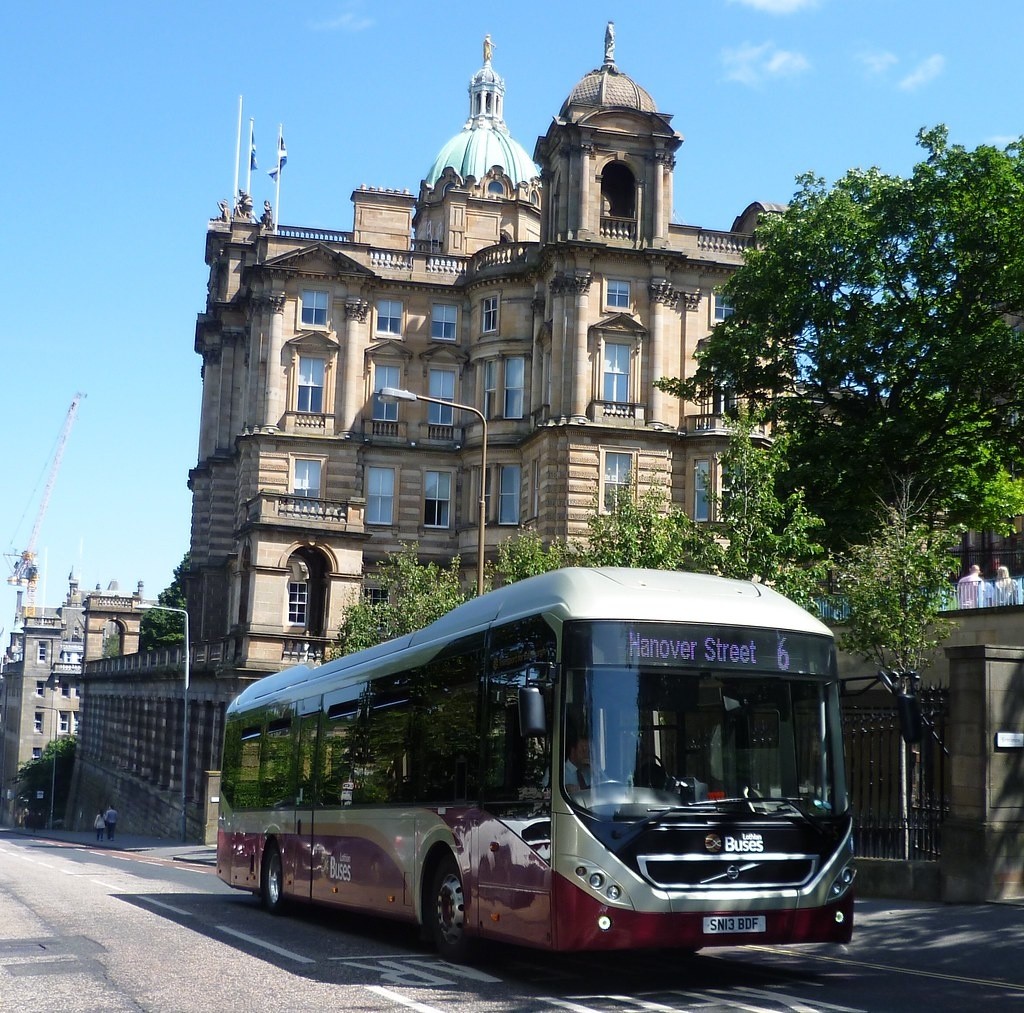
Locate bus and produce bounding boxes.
[216,567,928,968]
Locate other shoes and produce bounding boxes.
[110,839,114,841]
[96,839,99,841]
[101,839,103,842]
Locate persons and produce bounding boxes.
[233,188,254,219]
[258,200,273,231]
[94,804,118,841]
[209,199,232,222]
[565,733,613,794]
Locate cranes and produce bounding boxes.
[6,390,88,611]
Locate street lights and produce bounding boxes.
[36,705,59,832]
[133,603,188,844]
[376,386,488,596]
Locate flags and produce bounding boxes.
[250,130,259,171]
[267,135,288,182]
